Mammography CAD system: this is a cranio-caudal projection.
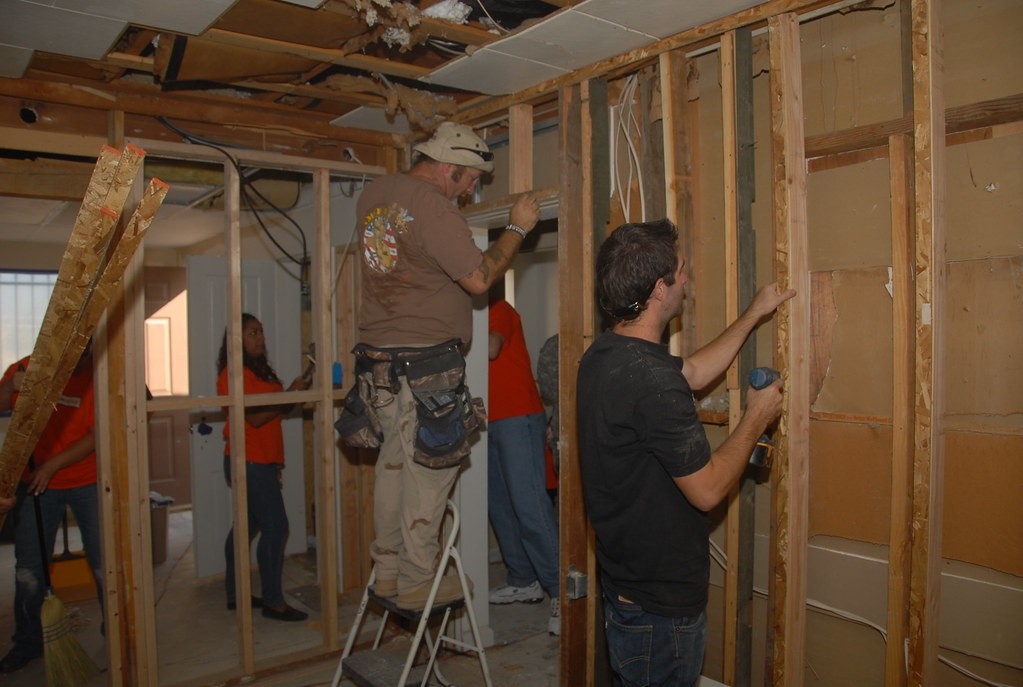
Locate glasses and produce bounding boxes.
[451,146,493,163]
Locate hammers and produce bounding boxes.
[302,343,315,378]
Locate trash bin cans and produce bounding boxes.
[151,502,168,563]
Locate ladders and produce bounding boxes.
[331,499,493,687]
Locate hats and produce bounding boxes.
[412,121,494,174]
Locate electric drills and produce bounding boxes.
[746,367,781,485]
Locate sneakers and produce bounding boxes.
[377,577,397,598]
[397,574,474,610]
[548,597,561,635]
[489,580,544,604]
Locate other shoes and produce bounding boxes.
[0,642,45,674]
[261,603,309,622]
[229,596,260,610]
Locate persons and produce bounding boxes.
[0,336,106,674]
[216,313,309,621]
[350,121,542,613]
[537,334,559,475]
[576,218,797,687]
[488,297,559,636]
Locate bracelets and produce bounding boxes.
[504,223,527,239]
